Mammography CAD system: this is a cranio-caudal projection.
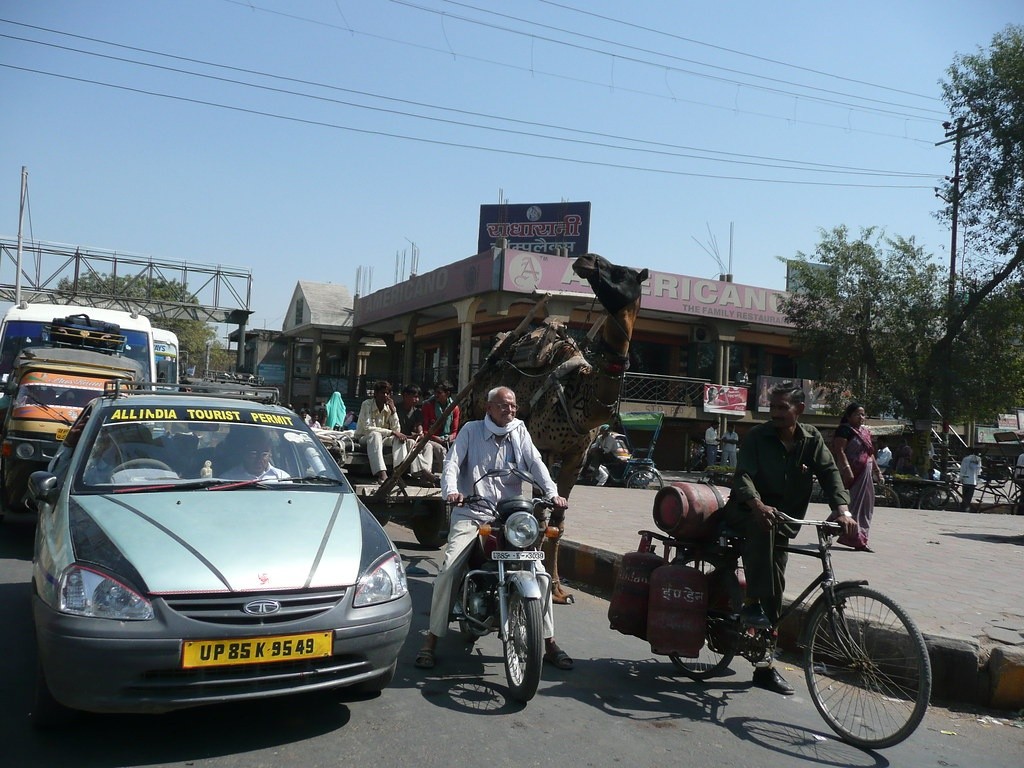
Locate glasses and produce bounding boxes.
[248,450,271,460]
[490,402,519,412]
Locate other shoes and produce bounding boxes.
[855,545,875,552]
[377,478,385,485]
[420,482,435,487]
[400,474,421,485]
[427,474,441,483]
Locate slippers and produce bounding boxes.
[415,648,435,668]
[544,650,574,669]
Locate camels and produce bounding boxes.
[454,254,649,606]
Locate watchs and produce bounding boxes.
[838,510,852,517]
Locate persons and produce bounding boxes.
[874,437,917,475]
[1013,453,1024,515]
[735,367,749,385]
[217,429,291,484]
[414,387,575,670]
[710,381,859,695]
[288,402,354,430]
[353,380,411,486]
[824,403,885,553]
[708,386,727,406]
[582,424,617,476]
[958,447,982,512]
[705,420,739,469]
[81,425,132,485]
[395,381,460,486]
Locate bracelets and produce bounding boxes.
[842,465,850,470]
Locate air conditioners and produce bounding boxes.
[689,325,715,343]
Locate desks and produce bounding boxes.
[879,476,956,510]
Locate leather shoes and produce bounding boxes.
[741,603,769,629]
[753,667,795,694]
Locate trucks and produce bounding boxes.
[0,344,144,520]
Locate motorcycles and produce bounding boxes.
[441,467,568,702]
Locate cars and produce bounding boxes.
[23,374,415,731]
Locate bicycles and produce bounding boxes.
[872,460,1024,516]
[666,504,934,750]
[578,425,665,491]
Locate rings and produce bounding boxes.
[400,438,404,440]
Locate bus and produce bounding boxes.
[0,298,181,397]
